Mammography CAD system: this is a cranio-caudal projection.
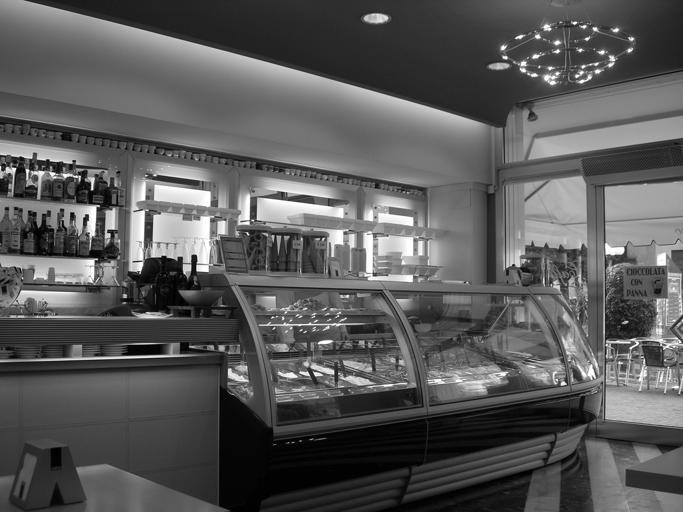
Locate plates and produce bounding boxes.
[0,345,128,360]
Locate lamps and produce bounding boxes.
[495,1,639,89]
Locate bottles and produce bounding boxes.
[188,254,201,290]
[172,256,188,318]
[0,151,127,206]
[0,206,119,260]
[154,256,172,315]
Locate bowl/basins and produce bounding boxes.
[134,199,241,220]
[385,252,403,275]
[372,255,392,275]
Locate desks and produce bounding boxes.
[622,437,683,495]
[0,464,227,512]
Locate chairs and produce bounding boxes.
[607,335,682,398]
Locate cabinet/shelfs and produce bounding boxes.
[370,229,444,280]
[192,267,607,512]
[0,189,123,292]
[249,215,358,278]
[131,202,240,270]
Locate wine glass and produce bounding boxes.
[92,263,119,287]
[135,241,178,260]
[176,237,218,265]
